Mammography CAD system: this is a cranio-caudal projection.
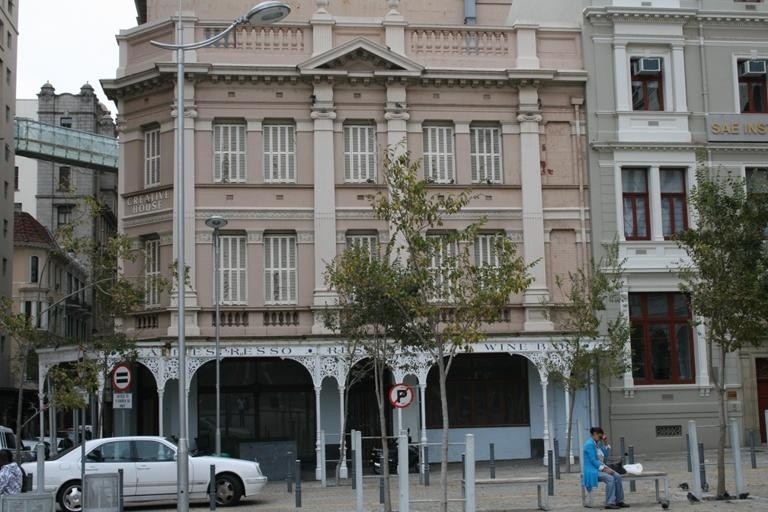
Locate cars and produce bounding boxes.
[23,426,90,464]
[20,436,268,511]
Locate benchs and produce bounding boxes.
[578,468,671,511]
[461,475,550,512]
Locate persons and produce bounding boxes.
[581,425,631,510]
[0,448,26,495]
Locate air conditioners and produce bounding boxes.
[739,59,766,76]
[633,58,662,76]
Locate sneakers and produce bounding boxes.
[616,502,630,507]
[605,504,619,509]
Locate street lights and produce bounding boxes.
[204,215,229,456]
[150,1,293,507]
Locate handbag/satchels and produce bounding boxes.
[607,462,626,474]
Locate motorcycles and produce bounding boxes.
[372,427,431,475]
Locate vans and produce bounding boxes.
[0,426,32,465]
